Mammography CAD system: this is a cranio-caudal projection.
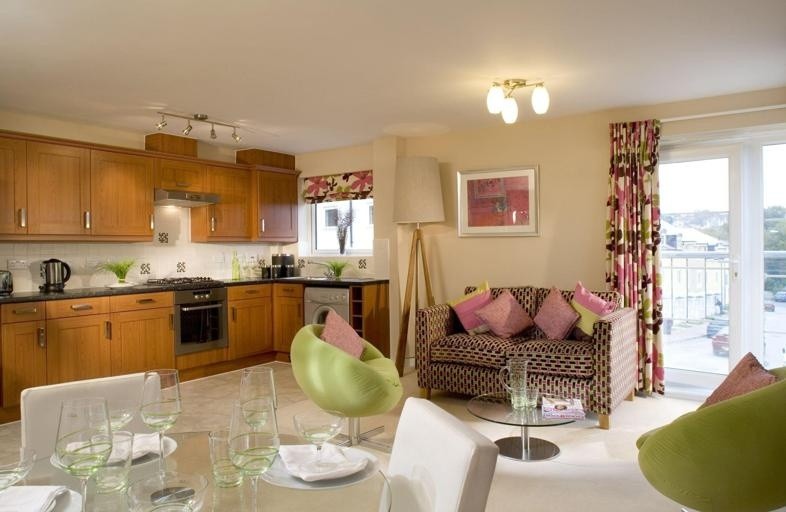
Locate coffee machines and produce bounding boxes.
[39,258,71,293]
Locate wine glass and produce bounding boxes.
[140,367,184,483]
[240,366,279,430]
[56,396,112,512]
[226,401,279,511]
[294,409,347,474]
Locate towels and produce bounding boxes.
[278,444,369,483]
[65,433,165,464]
[0,486,66,512]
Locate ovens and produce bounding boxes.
[174,302,229,357]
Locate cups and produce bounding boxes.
[94,428,134,496]
[499,357,528,409]
[207,426,243,487]
[524,389,539,409]
[121,470,209,512]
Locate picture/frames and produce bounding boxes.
[458,165,541,238]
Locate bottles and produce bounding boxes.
[231,247,241,282]
[244,252,295,279]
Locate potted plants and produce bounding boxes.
[326,261,350,280]
[93,260,139,287]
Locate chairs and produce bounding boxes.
[292,323,403,446]
[20,372,161,459]
[639,365,786,512]
[378,395,500,512]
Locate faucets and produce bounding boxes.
[307,260,331,277]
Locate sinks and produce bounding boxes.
[305,275,329,281]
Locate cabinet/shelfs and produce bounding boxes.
[0,132,300,245]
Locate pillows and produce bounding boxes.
[449,278,615,342]
[706,350,781,404]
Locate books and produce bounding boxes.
[541,396,586,421]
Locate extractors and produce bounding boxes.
[155,188,216,210]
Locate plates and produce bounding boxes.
[49,432,177,471]
[105,283,135,291]
[262,443,382,491]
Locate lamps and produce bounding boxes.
[393,154,447,377]
[487,78,549,124]
[155,111,243,142]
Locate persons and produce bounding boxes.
[546,396,574,410]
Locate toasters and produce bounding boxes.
[0,269,15,295]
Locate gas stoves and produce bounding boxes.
[145,276,225,305]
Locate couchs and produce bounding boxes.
[416,286,639,429]
